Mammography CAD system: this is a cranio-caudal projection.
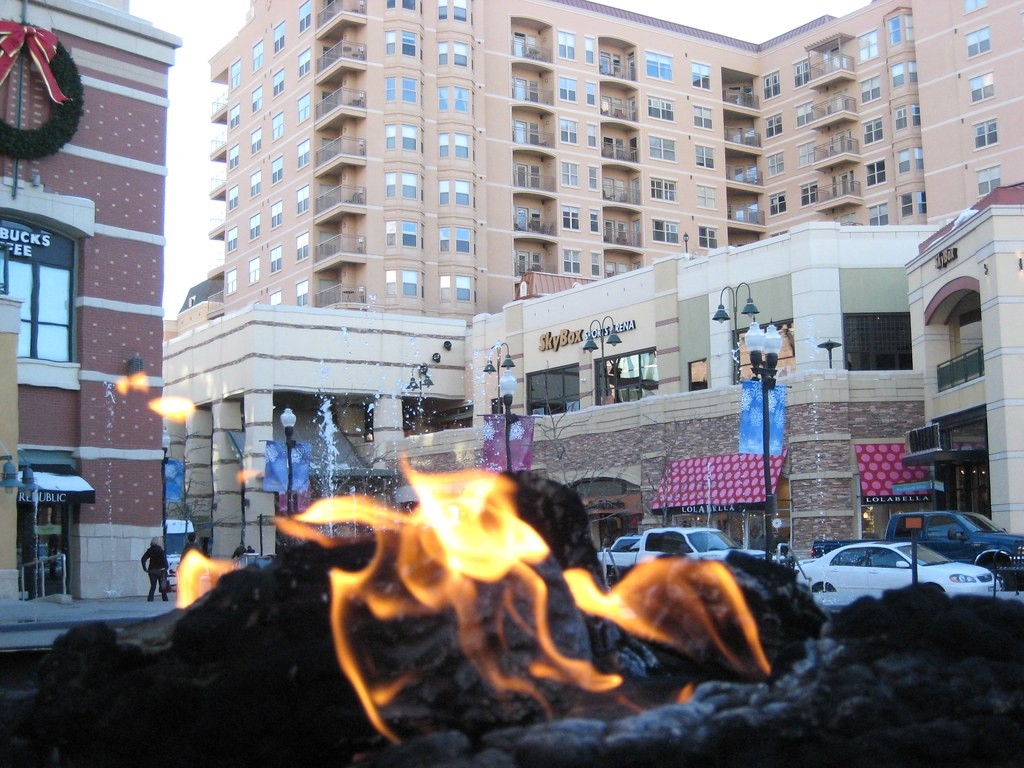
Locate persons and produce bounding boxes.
[142,536,169,602]
[232,541,254,559]
[182,533,200,560]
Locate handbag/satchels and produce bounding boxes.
[159,572,171,593]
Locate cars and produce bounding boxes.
[788,541,1001,618]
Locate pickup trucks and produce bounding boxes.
[595,525,765,589]
[807,509,1024,591]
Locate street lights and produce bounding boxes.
[279,407,298,520]
[744,322,783,612]
[498,368,516,474]
[161,428,172,557]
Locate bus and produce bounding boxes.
[164,520,199,594]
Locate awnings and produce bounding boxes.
[16,471,95,506]
[855,443,932,506]
[650,449,789,515]
